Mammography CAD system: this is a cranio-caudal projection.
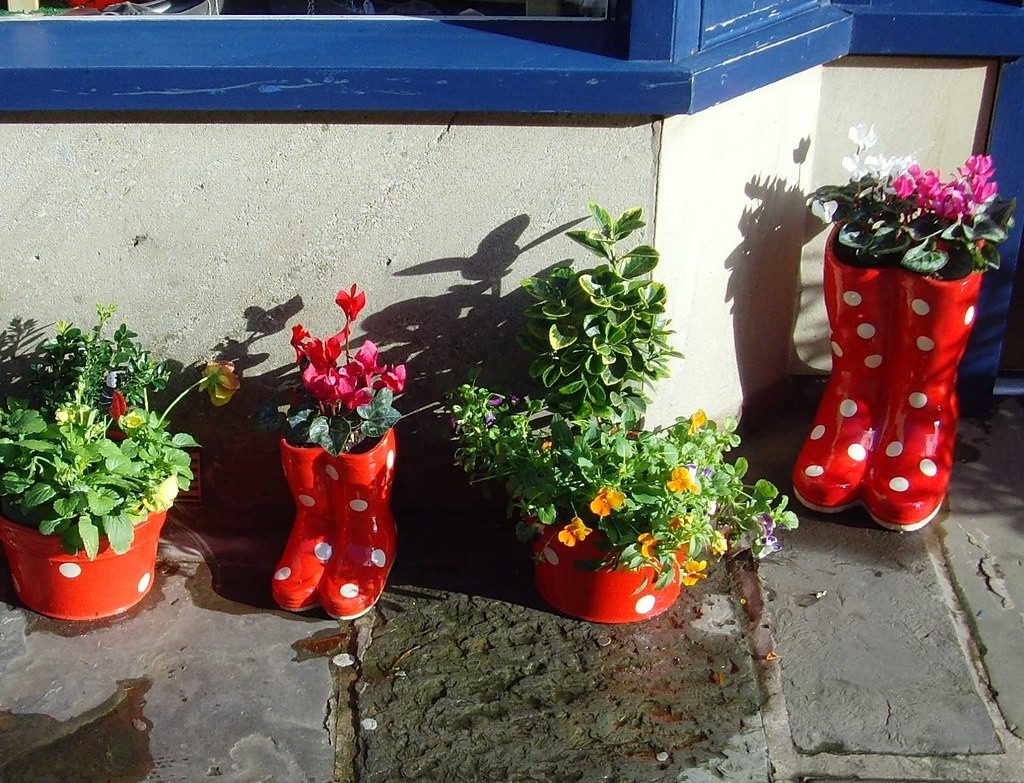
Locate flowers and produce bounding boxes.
[1,303,241,556]
[838,153,1018,283]
[438,198,801,586]
[278,286,411,457]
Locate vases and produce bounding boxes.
[525,519,688,626]
[268,428,396,619]
[790,226,983,531]
[0,512,163,622]
